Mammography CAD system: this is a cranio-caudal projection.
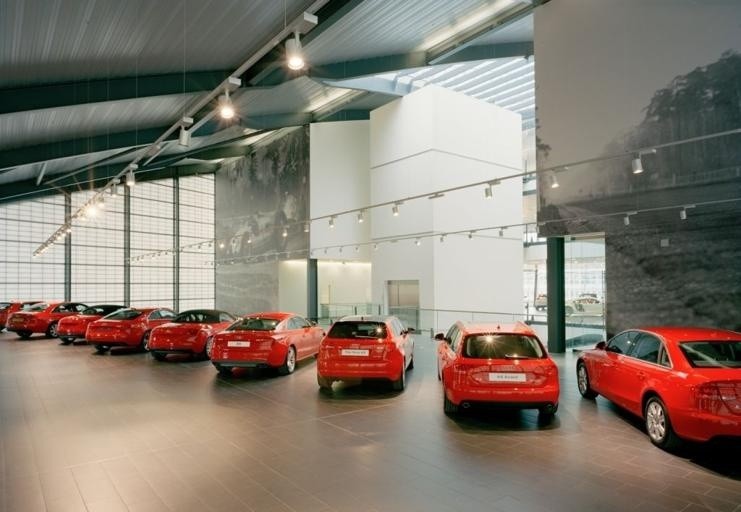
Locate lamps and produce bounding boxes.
[328,215,338,229]
[484,180,501,199]
[629,153,644,175]
[358,209,365,224]
[548,166,569,188]
[304,219,311,233]
[109,167,135,197]
[680,204,696,220]
[498,227,508,236]
[392,202,404,215]
[177,28,302,147]
[624,212,637,225]
[468,231,475,238]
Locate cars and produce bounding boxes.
[57,304,130,344]
[210,312,327,376]
[577,325,741,449]
[316,316,415,391]
[534,292,604,317]
[5,302,98,338]
[435,322,561,419]
[148,309,239,361]
[0,301,46,331]
[85,306,176,351]
